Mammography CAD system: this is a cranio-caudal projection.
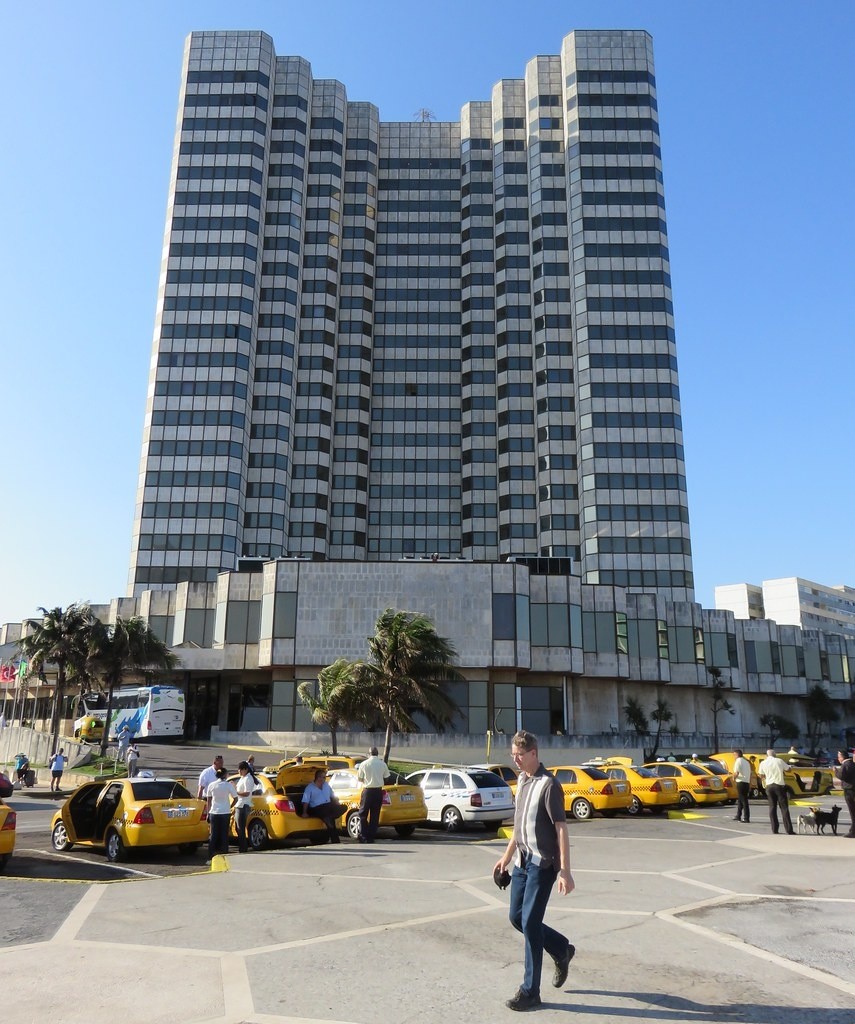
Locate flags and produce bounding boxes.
[19,660,28,677]
[1,664,16,682]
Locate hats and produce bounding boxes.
[122,726,129,729]
[18,753,26,755]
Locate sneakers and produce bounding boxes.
[552,945,575,988]
[505,991,541,1011]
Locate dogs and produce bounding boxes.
[797,807,820,834]
[809,804,842,835]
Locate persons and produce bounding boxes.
[689,753,698,763]
[127,740,140,778]
[354,746,391,844]
[294,756,303,766]
[14,753,28,785]
[227,759,255,853]
[206,767,237,865]
[245,754,255,772]
[116,725,130,763]
[732,749,753,822]
[493,730,576,1011]
[49,748,69,791]
[301,770,348,844]
[667,752,677,762]
[196,755,224,819]
[746,743,855,838]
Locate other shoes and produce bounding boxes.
[55,787,61,792]
[367,836,376,843]
[787,832,796,834]
[330,834,341,843]
[50,787,53,792]
[773,831,778,834]
[733,818,740,821]
[843,832,855,838]
[322,816,335,830]
[738,820,749,823]
[357,834,368,843]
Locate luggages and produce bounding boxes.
[26,766,35,787]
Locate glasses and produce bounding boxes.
[509,747,533,757]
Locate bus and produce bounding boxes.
[70,685,186,738]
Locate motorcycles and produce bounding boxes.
[79,716,104,745]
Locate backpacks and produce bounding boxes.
[845,762,855,785]
[49,754,64,769]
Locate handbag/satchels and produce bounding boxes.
[135,745,140,757]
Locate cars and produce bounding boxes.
[0,773,17,855]
[403,764,521,834]
[50,778,210,863]
[642,753,834,808]
[595,756,681,816]
[545,766,633,820]
[223,752,428,850]
